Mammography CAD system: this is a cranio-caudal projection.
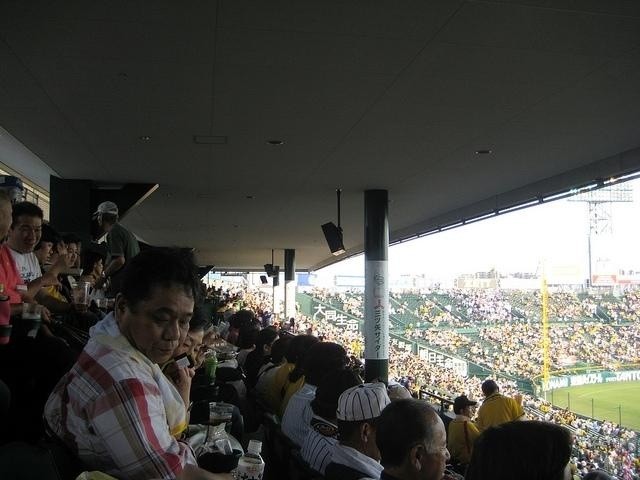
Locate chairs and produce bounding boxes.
[1,302,324,480]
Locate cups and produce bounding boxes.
[72,281,91,312]
[96,297,108,309]
[208,402,235,432]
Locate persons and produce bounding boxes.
[0,175,640,480]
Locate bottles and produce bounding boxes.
[235,439,266,480]
[202,351,218,385]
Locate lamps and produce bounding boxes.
[262,248,279,278]
[322,187,346,256]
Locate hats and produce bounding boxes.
[94,201,119,215]
[336,382,392,421]
[454,395,478,407]
